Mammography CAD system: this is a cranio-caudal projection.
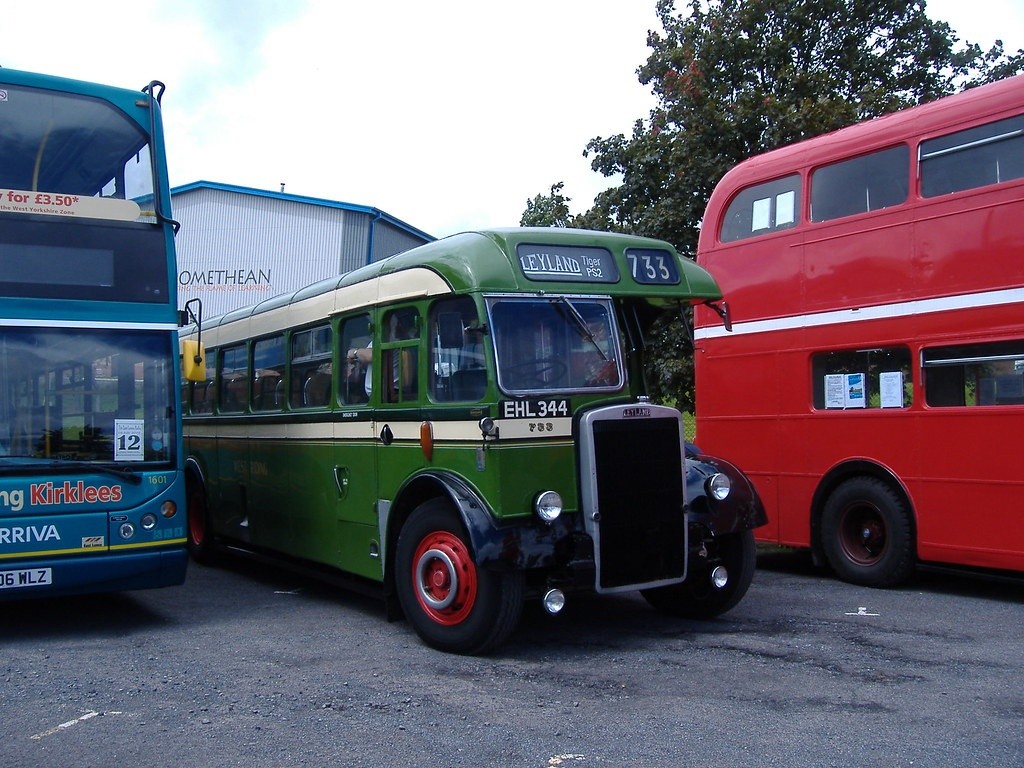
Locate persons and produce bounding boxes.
[347,314,414,404]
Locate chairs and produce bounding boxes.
[276,371,303,409]
[206,380,225,413]
[253,375,279,410]
[28,405,63,454]
[303,374,332,407]
[226,377,248,412]
[451,370,488,402]
[194,381,211,413]
[181,381,191,414]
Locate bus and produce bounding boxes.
[691,71,1024,594]
[41,377,147,451]
[153,228,770,655]
[0,66,207,614]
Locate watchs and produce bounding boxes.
[354,348,359,358]
